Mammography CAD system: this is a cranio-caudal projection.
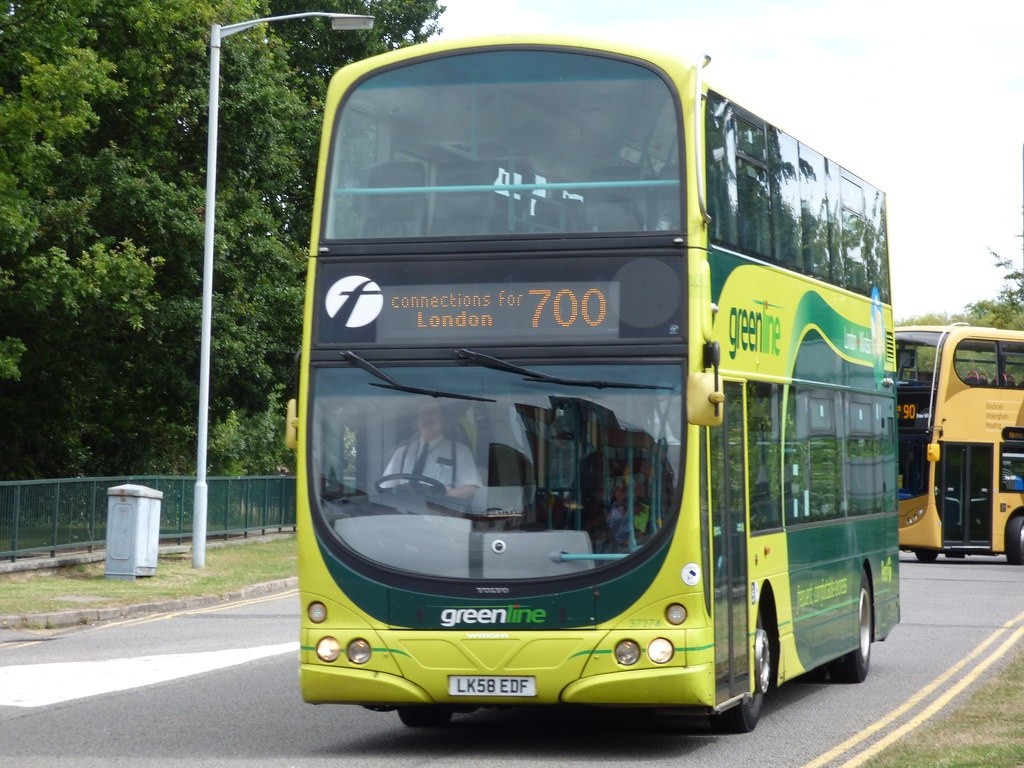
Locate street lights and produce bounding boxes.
[191,12,375,570]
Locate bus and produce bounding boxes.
[895,322,1024,563]
[296,35,901,733]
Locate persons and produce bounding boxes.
[379,398,482,501]
[566,450,676,553]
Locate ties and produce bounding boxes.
[409,442,430,482]
